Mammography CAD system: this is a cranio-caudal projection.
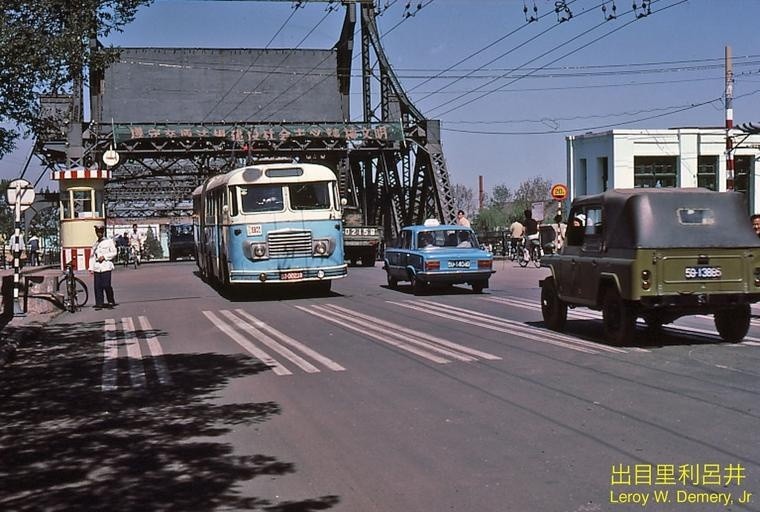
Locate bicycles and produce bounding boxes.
[503,234,544,268]
[119,244,142,269]
[56,256,88,313]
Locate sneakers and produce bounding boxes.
[92,301,116,309]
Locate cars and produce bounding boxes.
[382,218,496,295]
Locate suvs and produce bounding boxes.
[539,186,760,345]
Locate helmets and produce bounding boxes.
[93,224,105,233]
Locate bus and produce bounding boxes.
[191,162,349,296]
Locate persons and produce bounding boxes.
[9,227,25,269]
[118,231,130,247]
[419,234,435,249]
[750,214,760,237]
[509,217,524,254]
[456,231,473,248]
[26,232,41,267]
[128,223,143,259]
[87,224,117,309]
[574,217,583,227]
[458,210,470,228]
[521,209,539,262]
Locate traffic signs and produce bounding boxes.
[551,184,568,201]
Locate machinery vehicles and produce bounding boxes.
[340,205,382,267]
